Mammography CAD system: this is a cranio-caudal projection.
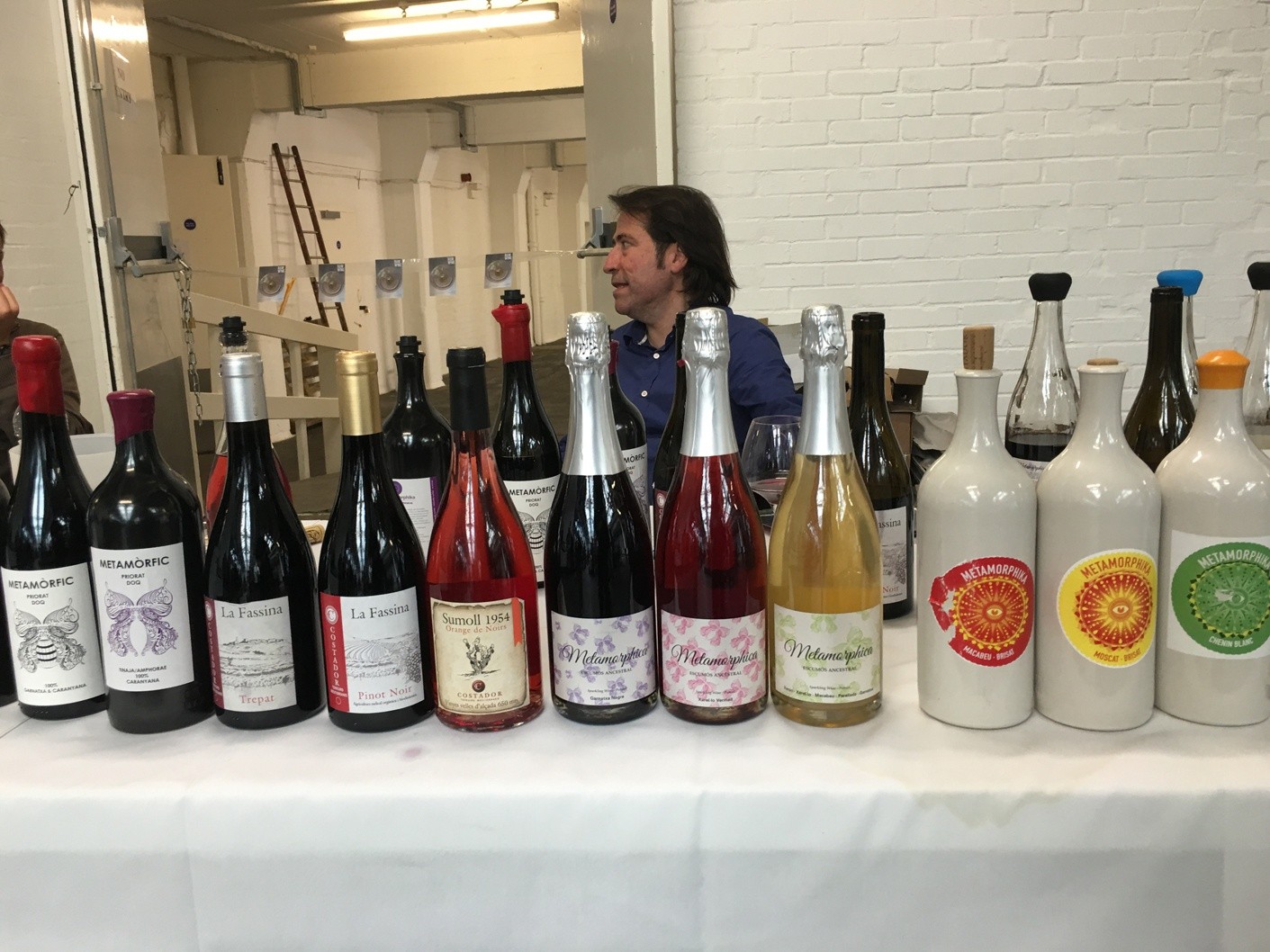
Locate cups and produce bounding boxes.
[9,433,117,491]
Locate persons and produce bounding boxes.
[559,184,797,502]
[0,224,95,447]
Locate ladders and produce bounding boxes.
[272,142,348,331]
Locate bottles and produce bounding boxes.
[849,311,914,620]
[1005,272,1080,485]
[205,316,293,539]
[543,312,659,726]
[1242,262,1270,460]
[490,290,562,589]
[1157,269,1204,417]
[1035,358,1160,731]
[0,335,109,721]
[200,353,328,731]
[659,307,768,726]
[1123,286,1196,473]
[1154,350,1270,726]
[85,389,216,734]
[917,327,1036,729]
[653,312,688,552]
[426,346,545,734]
[767,304,883,727]
[608,339,651,539]
[317,350,435,733]
[381,336,452,566]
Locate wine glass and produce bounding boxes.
[740,415,801,516]
